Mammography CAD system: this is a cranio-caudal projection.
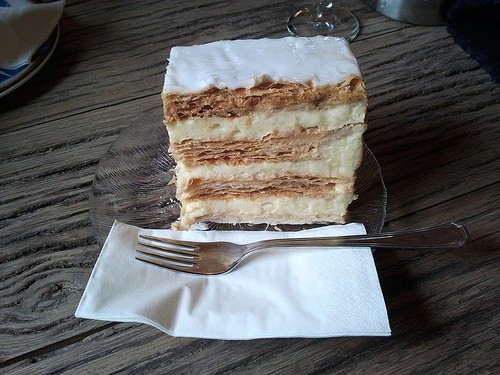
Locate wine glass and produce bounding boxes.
[286,0,360,43]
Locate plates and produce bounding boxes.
[89,106,388,254]
[0,0,59,98]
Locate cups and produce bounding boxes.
[366,0,449,26]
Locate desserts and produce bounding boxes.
[161,35,367,232]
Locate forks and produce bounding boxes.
[134,222,470,276]
